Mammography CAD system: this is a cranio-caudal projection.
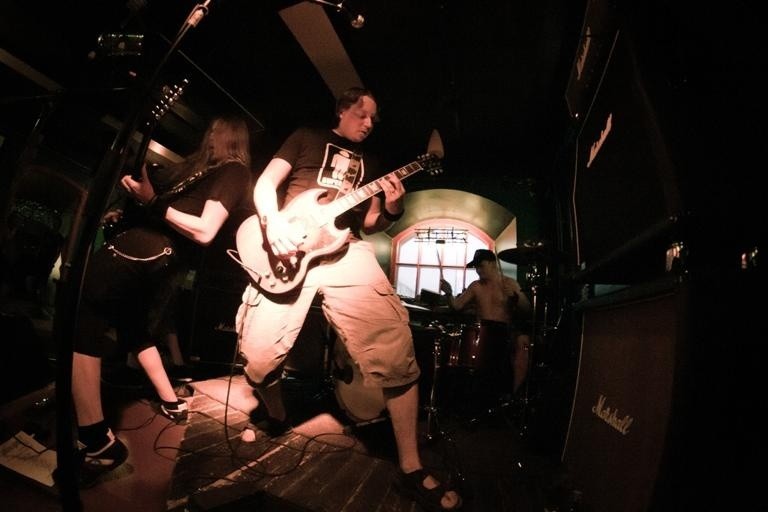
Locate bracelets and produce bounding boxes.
[381,200,404,223]
[145,194,157,210]
[509,293,520,303]
[148,197,170,221]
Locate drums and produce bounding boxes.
[448,320,507,373]
[331,335,387,424]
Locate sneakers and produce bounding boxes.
[159,399,188,425]
[52,428,128,491]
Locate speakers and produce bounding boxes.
[110,33,145,57]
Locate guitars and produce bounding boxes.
[226,152,445,295]
[102,76,189,278]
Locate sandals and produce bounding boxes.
[399,465,463,512]
[239,413,293,446]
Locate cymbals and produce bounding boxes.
[401,299,432,312]
[497,246,541,266]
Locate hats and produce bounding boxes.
[466,250,496,268]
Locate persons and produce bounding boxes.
[52,115,259,493]
[440,249,534,398]
[235,83,464,511]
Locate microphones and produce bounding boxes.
[186,1,212,30]
